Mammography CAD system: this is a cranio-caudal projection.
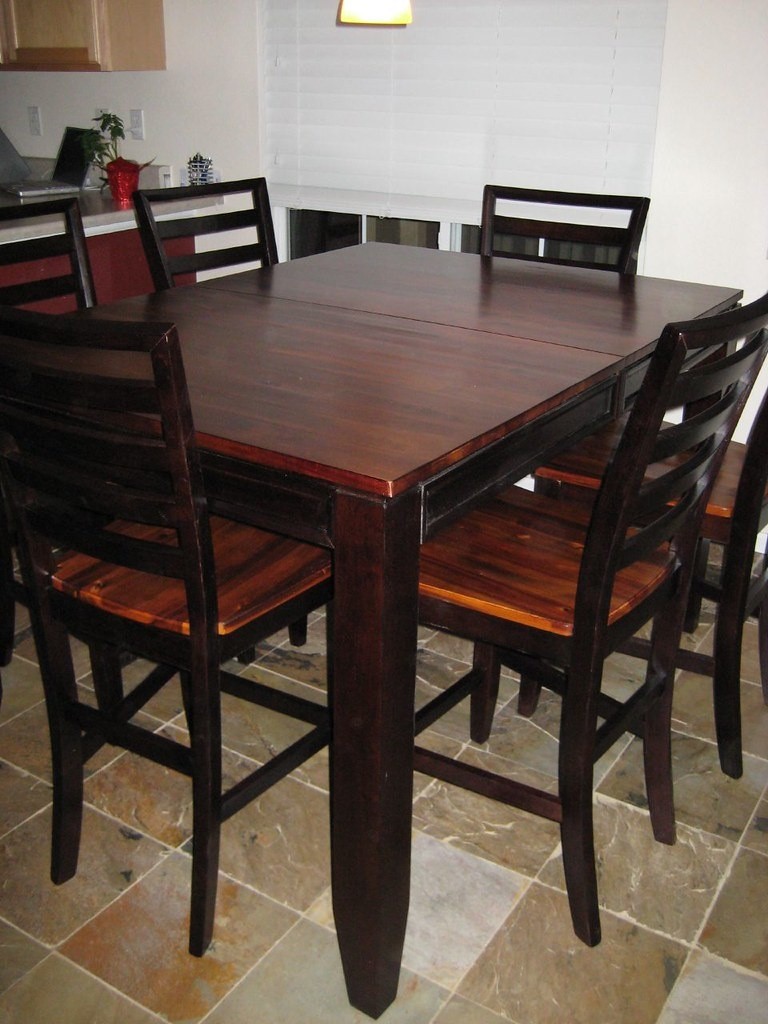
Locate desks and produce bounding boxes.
[1,241,743,1021]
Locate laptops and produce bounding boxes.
[0,127,100,196]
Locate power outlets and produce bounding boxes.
[28,105,42,136]
[130,109,144,140]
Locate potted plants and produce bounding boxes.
[80,112,154,201]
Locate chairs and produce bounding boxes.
[1,178,333,961]
[412,292,768,946]
[481,185,651,272]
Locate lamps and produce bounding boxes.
[339,1,412,23]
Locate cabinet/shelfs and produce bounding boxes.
[0,0,166,72]
[2,227,198,313]
